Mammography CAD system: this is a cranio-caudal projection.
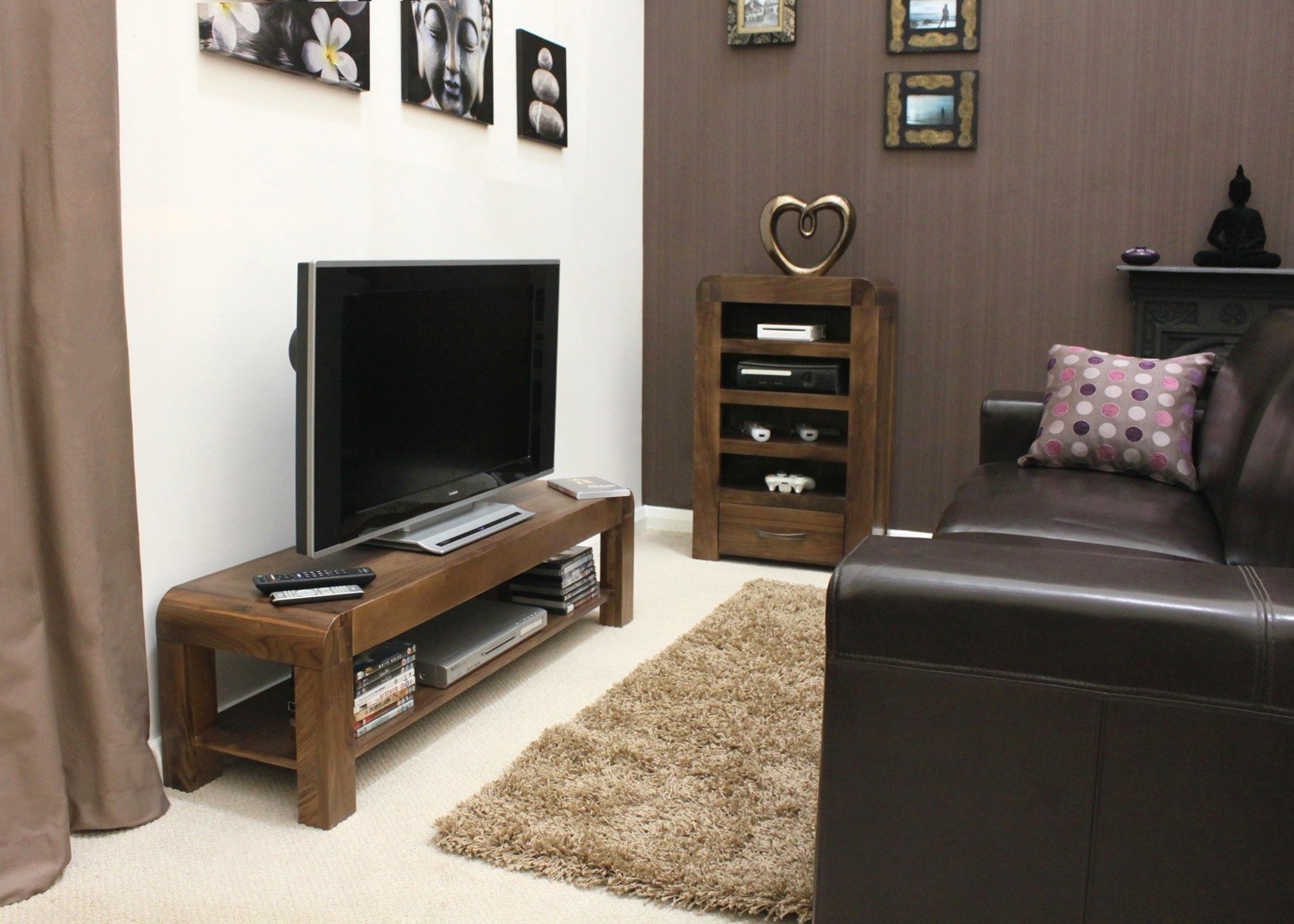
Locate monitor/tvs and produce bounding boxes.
[288,258,561,560]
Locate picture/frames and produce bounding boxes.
[886,0,980,55]
[885,69,979,151]
[728,0,797,47]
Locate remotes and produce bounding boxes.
[252,566,377,608]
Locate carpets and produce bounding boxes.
[433,580,827,924]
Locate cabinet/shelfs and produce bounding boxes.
[155,483,633,831]
[696,273,895,569]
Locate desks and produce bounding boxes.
[1117,264,1294,362]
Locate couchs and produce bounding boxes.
[817,309,1293,924]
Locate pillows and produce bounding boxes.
[1021,344,1216,492]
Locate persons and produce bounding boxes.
[938,3,950,28]
[1193,165,1282,269]
